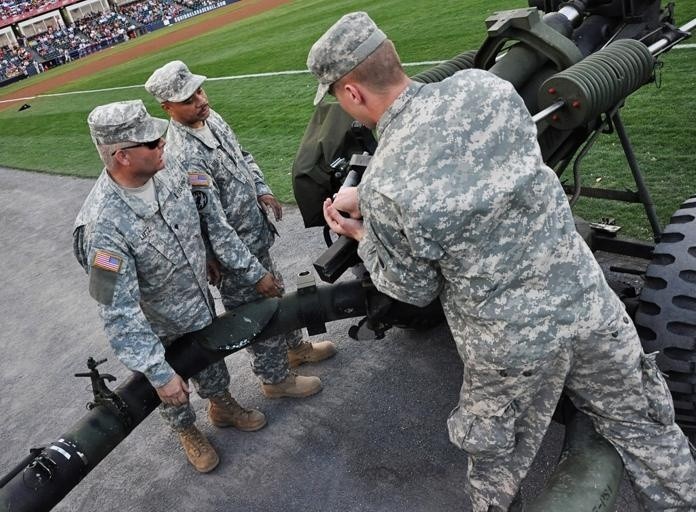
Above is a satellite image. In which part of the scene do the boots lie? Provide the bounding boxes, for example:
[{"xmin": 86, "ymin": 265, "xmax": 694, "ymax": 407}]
[
  {"xmin": 209, "ymin": 389, "xmax": 267, "ymax": 433},
  {"xmin": 287, "ymin": 339, "xmax": 337, "ymax": 368},
  {"xmin": 263, "ymin": 370, "xmax": 323, "ymax": 400},
  {"xmin": 180, "ymin": 424, "xmax": 220, "ymax": 474}
]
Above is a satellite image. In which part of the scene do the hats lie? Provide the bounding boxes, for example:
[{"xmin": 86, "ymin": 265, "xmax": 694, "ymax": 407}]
[
  {"xmin": 87, "ymin": 99, "xmax": 170, "ymax": 144},
  {"xmin": 144, "ymin": 60, "xmax": 208, "ymax": 103},
  {"xmin": 305, "ymin": 11, "xmax": 387, "ymax": 108}
]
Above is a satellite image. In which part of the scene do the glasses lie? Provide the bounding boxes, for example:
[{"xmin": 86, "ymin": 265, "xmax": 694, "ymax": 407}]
[{"xmin": 111, "ymin": 137, "xmax": 161, "ymax": 158}]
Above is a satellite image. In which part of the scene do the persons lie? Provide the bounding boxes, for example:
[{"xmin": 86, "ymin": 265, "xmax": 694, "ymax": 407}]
[
  {"xmin": 306, "ymin": 11, "xmax": 696, "ymax": 512},
  {"xmin": 72, "ymin": 99, "xmax": 267, "ymax": 473},
  {"xmin": 145, "ymin": 60, "xmax": 337, "ymax": 399},
  {"xmin": 0, "ymin": 0, "xmax": 224, "ymax": 82}
]
